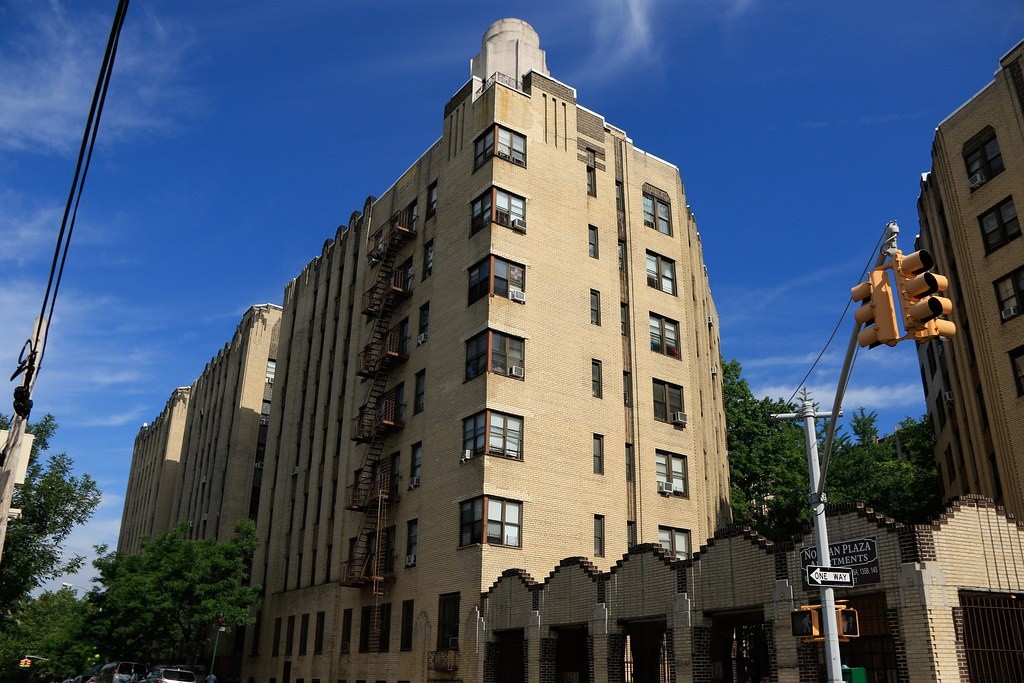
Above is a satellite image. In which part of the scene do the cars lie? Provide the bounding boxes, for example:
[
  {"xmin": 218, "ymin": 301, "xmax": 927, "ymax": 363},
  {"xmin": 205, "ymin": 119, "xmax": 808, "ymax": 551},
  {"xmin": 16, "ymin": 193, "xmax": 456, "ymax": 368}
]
[
  {"xmin": 137, "ymin": 667, "xmax": 197, "ymax": 683},
  {"xmin": 61, "ymin": 675, "xmax": 94, "ymax": 683}
]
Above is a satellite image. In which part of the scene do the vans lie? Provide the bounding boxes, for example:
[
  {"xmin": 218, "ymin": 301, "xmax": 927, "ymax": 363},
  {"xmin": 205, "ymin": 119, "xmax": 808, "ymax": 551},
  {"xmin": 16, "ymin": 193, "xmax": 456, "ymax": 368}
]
[{"xmin": 95, "ymin": 661, "xmax": 148, "ymax": 683}]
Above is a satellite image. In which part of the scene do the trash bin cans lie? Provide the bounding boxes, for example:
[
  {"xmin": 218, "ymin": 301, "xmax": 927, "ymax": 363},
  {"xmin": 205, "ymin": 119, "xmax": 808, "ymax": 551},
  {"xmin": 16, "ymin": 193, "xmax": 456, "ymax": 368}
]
[{"xmin": 842, "ymin": 668, "xmax": 867, "ymax": 683}]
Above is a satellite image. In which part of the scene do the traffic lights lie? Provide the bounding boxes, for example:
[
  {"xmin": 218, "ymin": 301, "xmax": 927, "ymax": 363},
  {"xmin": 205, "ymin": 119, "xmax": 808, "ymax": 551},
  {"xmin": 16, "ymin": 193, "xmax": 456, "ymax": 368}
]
[
  {"xmin": 27, "ymin": 660, "xmax": 31, "ymax": 667},
  {"xmin": 790, "ymin": 608, "xmax": 819, "ymax": 638},
  {"xmin": 926, "ymin": 269, "xmax": 957, "ymax": 337},
  {"xmin": 889, "ymin": 249, "xmax": 943, "ymax": 341},
  {"xmin": 19, "ymin": 660, "xmax": 25, "ymax": 667},
  {"xmin": 23, "ymin": 659, "xmax": 28, "ymax": 667},
  {"xmin": 851, "ymin": 269, "xmax": 899, "ymax": 346},
  {"xmin": 837, "ymin": 607, "xmax": 860, "ymax": 638}
]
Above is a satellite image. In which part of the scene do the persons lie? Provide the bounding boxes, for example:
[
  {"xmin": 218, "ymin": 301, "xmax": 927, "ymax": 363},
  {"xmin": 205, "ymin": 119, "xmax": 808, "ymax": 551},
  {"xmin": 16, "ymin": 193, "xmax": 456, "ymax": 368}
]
[{"xmin": 205, "ymin": 672, "xmax": 216, "ymax": 683}]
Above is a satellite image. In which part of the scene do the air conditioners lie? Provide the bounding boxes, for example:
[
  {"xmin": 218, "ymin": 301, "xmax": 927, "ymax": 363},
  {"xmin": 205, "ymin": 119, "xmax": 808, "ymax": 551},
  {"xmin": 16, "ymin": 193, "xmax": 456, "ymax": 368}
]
[
  {"xmin": 708, "ymin": 316, "xmax": 714, "ymax": 326},
  {"xmin": 511, "ymin": 290, "xmax": 526, "ymax": 302},
  {"xmin": 405, "ymin": 555, "xmax": 416, "ymax": 566},
  {"xmin": 260, "ymin": 419, "xmax": 266, "ymax": 424},
  {"xmin": 509, "ymin": 365, "xmax": 524, "ymax": 378},
  {"xmin": 201, "ymin": 513, "xmax": 208, "ymax": 520},
  {"xmin": 200, "ymin": 476, "xmax": 206, "ymax": 483},
  {"xmin": 450, "ymin": 637, "xmax": 457, "ymax": 649},
  {"xmin": 187, "ymin": 521, "xmax": 194, "ymax": 527},
  {"xmin": 511, "ymin": 219, "xmax": 527, "ymax": 230},
  {"xmin": 410, "ymin": 477, "xmax": 420, "ymax": 487},
  {"xmin": 199, "ymin": 409, "xmax": 204, "ymax": 415},
  {"xmin": 291, "ymin": 466, "xmax": 298, "ymax": 475},
  {"xmin": 712, "ymin": 366, "xmax": 717, "ymax": 375},
  {"xmin": 268, "ymin": 377, "xmax": 274, "ymax": 383},
  {"xmin": 1001, "ymin": 307, "xmax": 1018, "ymax": 319},
  {"xmin": 460, "ymin": 449, "xmax": 471, "ymax": 461},
  {"xmin": 671, "ymin": 412, "xmax": 688, "ymax": 425},
  {"xmin": 968, "ymin": 174, "xmax": 986, "ymax": 188},
  {"xmin": 417, "ymin": 333, "xmax": 427, "ymax": 344},
  {"xmin": 257, "ymin": 462, "xmax": 263, "ymax": 469},
  {"xmin": 937, "ymin": 345, "xmax": 943, "ymax": 355},
  {"xmin": 658, "ymin": 482, "xmax": 674, "ymax": 497},
  {"xmin": 943, "ymin": 391, "xmax": 953, "ymax": 403}
]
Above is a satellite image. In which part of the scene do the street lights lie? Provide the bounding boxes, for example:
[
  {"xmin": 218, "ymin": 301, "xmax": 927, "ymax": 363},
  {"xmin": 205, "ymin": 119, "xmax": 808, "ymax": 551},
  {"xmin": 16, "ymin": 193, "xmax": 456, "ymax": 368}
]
[{"xmin": 209, "ymin": 625, "xmax": 229, "ymax": 674}]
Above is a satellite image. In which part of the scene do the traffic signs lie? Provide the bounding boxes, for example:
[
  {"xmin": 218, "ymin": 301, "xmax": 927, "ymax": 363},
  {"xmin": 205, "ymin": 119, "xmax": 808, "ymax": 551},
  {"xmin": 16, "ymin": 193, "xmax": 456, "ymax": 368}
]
[{"xmin": 804, "ymin": 564, "xmax": 855, "ymax": 588}]
